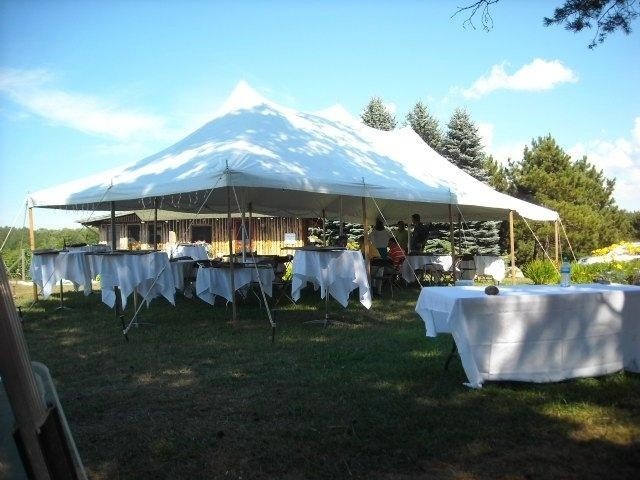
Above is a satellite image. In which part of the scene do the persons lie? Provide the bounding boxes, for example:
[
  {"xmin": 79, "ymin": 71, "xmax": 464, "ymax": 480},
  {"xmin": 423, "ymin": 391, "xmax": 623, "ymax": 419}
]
[
  {"xmin": 393, "ymin": 220, "xmax": 408, "ymax": 252},
  {"xmin": 358, "ymin": 236, "xmax": 384, "ymax": 294},
  {"xmin": 410, "ymin": 214, "xmax": 427, "ymax": 252},
  {"xmin": 384, "ymin": 237, "xmax": 406, "ymax": 275},
  {"xmin": 369, "ymin": 220, "xmax": 393, "ymax": 254}
]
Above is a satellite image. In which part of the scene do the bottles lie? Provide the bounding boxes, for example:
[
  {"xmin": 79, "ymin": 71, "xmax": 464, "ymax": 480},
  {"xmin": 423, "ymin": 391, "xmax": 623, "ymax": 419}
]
[{"xmin": 560, "ymin": 258, "xmax": 571, "ymax": 287}]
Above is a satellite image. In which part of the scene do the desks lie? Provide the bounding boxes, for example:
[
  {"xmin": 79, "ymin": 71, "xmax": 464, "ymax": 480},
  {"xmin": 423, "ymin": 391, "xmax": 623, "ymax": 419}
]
[{"xmin": 29, "ymin": 241, "xmax": 505, "ymax": 322}]
